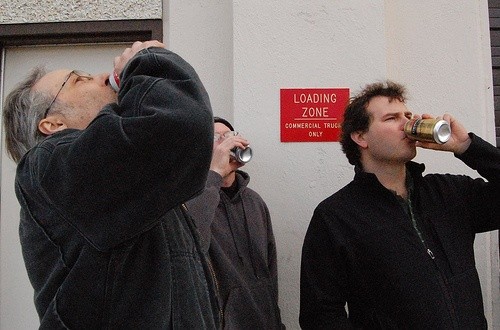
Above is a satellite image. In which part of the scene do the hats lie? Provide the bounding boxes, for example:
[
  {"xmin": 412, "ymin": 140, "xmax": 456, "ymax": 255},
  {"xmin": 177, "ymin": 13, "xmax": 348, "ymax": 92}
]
[{"xmin": 214, "ymin": 116, "xmax": 234, "ymax": 131}]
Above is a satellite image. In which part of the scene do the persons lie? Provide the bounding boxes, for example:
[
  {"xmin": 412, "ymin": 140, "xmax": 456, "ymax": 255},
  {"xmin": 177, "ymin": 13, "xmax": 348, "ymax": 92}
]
[
  {"xmin": 2, "ymin": 41, "xmax": 214, "ymax": 330},
  {"xmin": 182, "ymin": 116, "xmax": 286, "ymax": 330},
  {"xmin": 298, "ymin": 81, "xmax": 500, "ymax": 330}
]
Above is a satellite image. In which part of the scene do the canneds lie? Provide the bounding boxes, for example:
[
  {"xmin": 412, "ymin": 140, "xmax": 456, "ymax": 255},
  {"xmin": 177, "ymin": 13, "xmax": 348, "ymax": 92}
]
[
  {"xmin": 108, "ymin": 69, "xmax": 121, "ymax": 93},
  {"xmin": 229, "ymin": 145, "xmax": 253, "ymax": 164},
  {"xmin": 404, "ymin": 118, "xmax": 451, "ymax": 145}
]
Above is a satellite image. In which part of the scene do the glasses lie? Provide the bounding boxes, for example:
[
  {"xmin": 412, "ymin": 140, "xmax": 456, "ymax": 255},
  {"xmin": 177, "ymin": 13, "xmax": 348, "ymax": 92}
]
[
  {"xmin": 213, "ymin": 131, "xmax": 239, "ymax": 142},
  {"xmin": 44, "ymin": 70, "xmax": 93, "ymax": 118}
]
